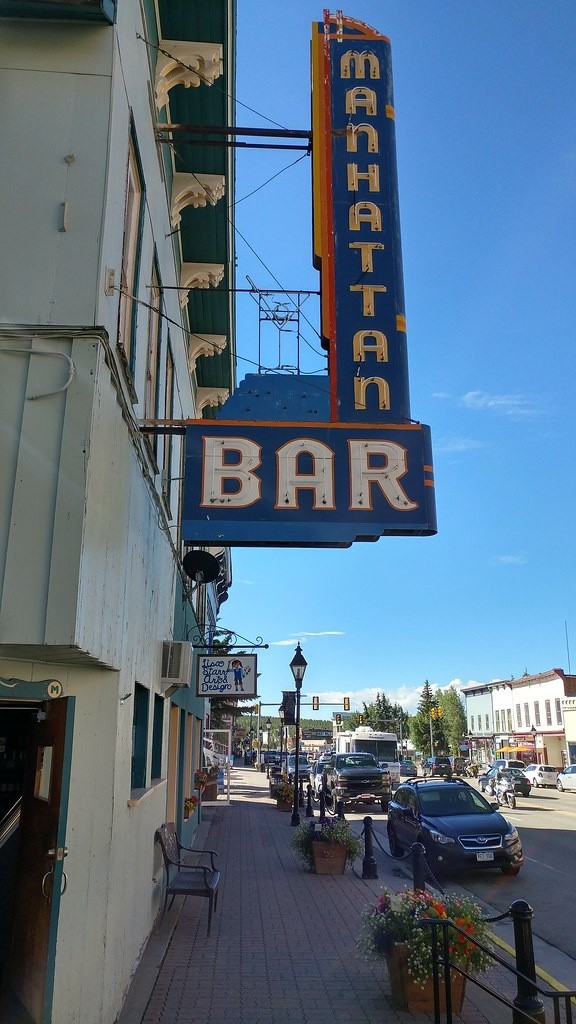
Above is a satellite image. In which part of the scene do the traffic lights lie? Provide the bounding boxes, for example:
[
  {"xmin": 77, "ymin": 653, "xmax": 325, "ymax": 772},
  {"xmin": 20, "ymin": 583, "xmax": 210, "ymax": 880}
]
[
  {"xmin": 358, "ymin": 714, "xmax": 363, "ymax": 725},
  {"xmin": 336, "ymin": 714, "xmax": 342, "ymax": 725},
  {"xmin": 431, "ymin": 705, "xmax": 443, "ymax": 719}
]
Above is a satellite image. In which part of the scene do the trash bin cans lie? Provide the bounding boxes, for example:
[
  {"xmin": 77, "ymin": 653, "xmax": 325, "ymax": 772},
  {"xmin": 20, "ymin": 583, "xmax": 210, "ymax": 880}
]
[{"xmin": 270, "ymin": 774, "xmax": 284, "ymax": 798}]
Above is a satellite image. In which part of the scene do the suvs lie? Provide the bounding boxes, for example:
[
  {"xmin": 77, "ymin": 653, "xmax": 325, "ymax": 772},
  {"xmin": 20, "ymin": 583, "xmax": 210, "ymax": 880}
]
[
  {"xmin": 321, "ymin": 753, "xmax": 392, "ymax": 812},
  {"xmin": 387, "ymin": 777, "xmax": 524, "ymax": 880},
  {"xmin": 489, "ymin": 759, "xmax": 527, "ymax": 774},
  {"xmin": 420, "ymin": 756, "xmax": 468, "ymax": 777}
]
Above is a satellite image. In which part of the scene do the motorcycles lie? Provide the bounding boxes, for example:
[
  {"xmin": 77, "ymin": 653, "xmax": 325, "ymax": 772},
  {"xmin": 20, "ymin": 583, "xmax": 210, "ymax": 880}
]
[{"xmin": 485, "ymin": 777, "xmax": 516, "ymax": 810}]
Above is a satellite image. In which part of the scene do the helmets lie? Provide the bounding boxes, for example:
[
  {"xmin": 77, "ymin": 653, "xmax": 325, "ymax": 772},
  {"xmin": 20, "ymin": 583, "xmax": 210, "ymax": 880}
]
[{"xmin": 499, "ymin": 765, "xmax": 506, "ymax": 772}]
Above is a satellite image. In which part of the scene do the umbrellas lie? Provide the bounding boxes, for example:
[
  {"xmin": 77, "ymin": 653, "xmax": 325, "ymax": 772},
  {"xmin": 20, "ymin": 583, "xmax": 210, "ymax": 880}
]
[{"xmin": 495, "ymin": 746, "xmax": 530, "ymax": 752}]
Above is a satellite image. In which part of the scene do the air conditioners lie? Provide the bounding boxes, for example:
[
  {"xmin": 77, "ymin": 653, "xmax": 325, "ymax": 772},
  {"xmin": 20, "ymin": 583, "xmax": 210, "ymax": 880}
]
[{"xmin": 159, "ymin": 641, "xmax": 193, "ymax": 687}]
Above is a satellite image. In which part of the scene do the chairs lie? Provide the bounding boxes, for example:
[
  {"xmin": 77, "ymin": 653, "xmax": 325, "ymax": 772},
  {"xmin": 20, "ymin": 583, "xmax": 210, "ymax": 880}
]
[{"xmin": 156, "ymin": 821, "xmax": 220, "ymax": 926}]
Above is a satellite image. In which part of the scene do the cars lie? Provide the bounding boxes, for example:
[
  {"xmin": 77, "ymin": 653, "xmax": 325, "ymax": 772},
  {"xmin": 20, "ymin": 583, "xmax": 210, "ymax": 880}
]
[
  {"xmin": 478, "ymin": 768, "xmax": 531, "ymax": 798},
  {"xmin": 522, "ymin": 764, "xmax": 559, "ymax": 788},
  {"xmin": 556, "ymin": 764, "xmax": 576, "ymax": 792},
  {"xmin": 247, "ymin": 748, "xmax": 338, "ymax": 798}
]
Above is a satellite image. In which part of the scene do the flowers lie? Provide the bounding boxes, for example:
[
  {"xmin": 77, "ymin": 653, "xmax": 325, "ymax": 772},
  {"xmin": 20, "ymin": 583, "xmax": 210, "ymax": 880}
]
[
  {"xmin": 206, "ymin": 766, "xmax": 219, "ymax": 782},
  {"xmin": 355, "ymin": 888, "xmax": 499, "ymax": 991},
  {"xmin": 184, "ymin": 796, "xmax": 200, "ymax": 810},
  {"xmin": 291, "ymin": 816, "xmax": 364, "ymax": 869},
  {"xmin": 272, "ymin": 783, "xmax": 295, "ymax": 803},
  {"xmin": 195, "ymin": 769, "xmax": 207, "ymax": 791}
]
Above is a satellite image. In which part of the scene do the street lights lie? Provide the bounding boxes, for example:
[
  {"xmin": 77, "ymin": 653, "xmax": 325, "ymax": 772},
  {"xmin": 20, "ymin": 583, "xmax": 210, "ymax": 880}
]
[
  {"xmin": 278, "ymin": 703, "xmax": 284, "ymax": 774},
  {"xmin": 530, "ymin": 725, "xmax": 537, "ymax": 764},
  {"xmin": 400, "ymin": 760, "xmax": 417, "ymax": 777},
  {"xmin": 289, "ymin": 642, "xmax": 308, "ymax": 826},
  {"xmin": 265, "ymin": 718, "xmax": 272, "ymax": 780},
  {"xmin": 468, "ymin": 730, "xmax": 473, "ymax": 762}
]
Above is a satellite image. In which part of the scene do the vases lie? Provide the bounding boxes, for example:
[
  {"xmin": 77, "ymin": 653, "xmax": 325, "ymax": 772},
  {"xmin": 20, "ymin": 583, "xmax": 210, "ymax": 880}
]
[
  {"xmin": 201, "ymin": 786, "xmax": 206, "ymax": 793},
  {"xmin": 206, "ymin": 780, "xmax": 216, "ymax": 786},
  {"xmin": 278, "ymin": 797, "xmax": 292, "ymax": 812},
  {"xmin": 185, "ymin": 808, "xmax": 194, "ymax": 819},
  {"xmin": 385, "ymin": 944, "xmax": 468, "ymax": 1013},
  {"xmin": 312, "ymin": 841, "xmax": 350, "ymax": 874}
]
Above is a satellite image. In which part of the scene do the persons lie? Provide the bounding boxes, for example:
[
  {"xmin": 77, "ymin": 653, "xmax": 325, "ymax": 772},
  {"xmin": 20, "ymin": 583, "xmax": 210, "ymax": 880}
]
[
  {"xmin": 233, "ymin": 747, "xmax": 240, "ymax": 760},
  {"xmin": 495, "ymin": 765, "xmax": 515, "ymax": 785}
]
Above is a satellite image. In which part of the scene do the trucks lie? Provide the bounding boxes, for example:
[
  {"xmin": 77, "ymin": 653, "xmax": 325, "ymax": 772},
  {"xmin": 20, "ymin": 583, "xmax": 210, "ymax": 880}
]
[{"xmin": 335, "ymin": 728, "xmax": 400, "ymax": 785}]
